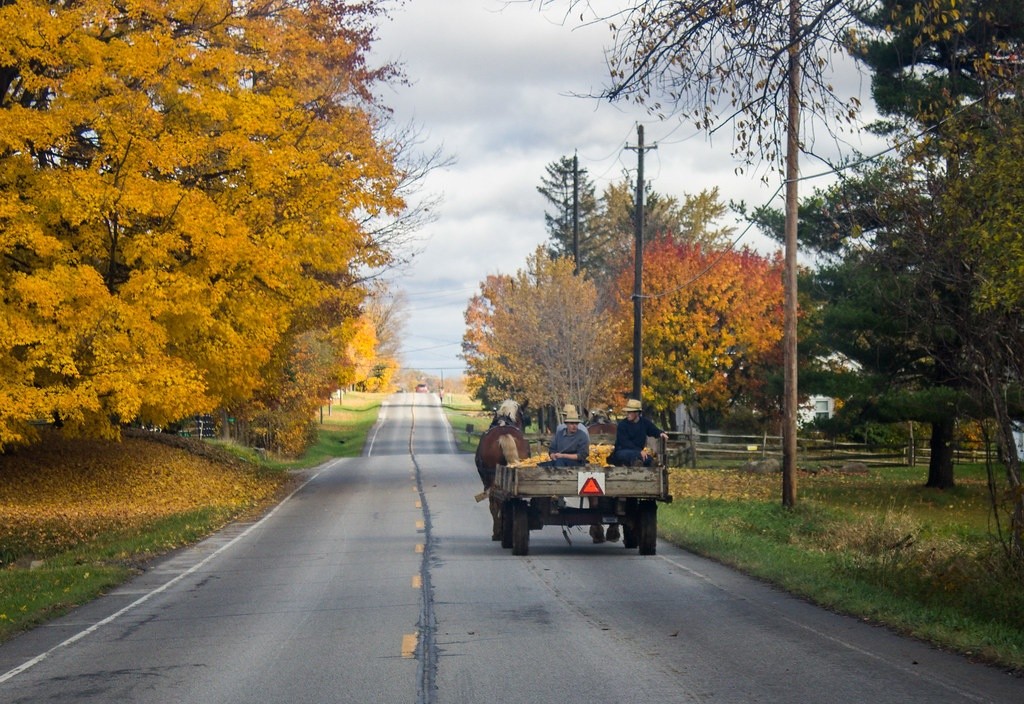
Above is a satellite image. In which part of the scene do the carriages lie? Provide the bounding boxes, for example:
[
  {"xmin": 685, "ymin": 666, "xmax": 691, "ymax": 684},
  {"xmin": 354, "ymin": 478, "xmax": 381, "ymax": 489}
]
[{"xmin": 474, "ymin": 398, "xmax": 675, "ymax": 556}]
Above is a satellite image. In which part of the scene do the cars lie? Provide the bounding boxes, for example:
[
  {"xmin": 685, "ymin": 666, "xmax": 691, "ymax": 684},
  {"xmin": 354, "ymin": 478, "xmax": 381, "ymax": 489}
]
[{"xmin": 416, "ymin": 383, "xmax": 428, "ymax": 394}]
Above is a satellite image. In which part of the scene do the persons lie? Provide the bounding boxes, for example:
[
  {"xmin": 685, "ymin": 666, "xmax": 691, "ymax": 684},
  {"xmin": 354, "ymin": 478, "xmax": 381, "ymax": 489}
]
[
  {"xmin": 613, "ymin": 399, "xmax": 669, "ymax": 466},
  {"xmin": 537, "ymin": 403, "xmax": 590, "ymax": 467}
]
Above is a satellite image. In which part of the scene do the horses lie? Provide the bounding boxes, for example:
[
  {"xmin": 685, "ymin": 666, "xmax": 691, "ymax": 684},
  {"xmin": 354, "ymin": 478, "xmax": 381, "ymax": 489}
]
[
  {"xmin": 474, "ymin": 398, "xmax": 532, "ymax": 542},
  {"xmin": 585, "ymin": 408, "xmax": 620, "ymax": 544}
]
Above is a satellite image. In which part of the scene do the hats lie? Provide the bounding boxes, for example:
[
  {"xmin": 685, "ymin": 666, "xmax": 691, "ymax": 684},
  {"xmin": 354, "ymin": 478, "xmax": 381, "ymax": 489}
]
[
  {"xmin": 558, "ymin": 405, "xmax": 575, "ymax": 415},
  {"xmin": 564, "ymin": 410, "xmax": 581, "ymax": 423},
  {"xmin": 621, "ymin": 399, "xmax": 642, "ymax": 411}
]
